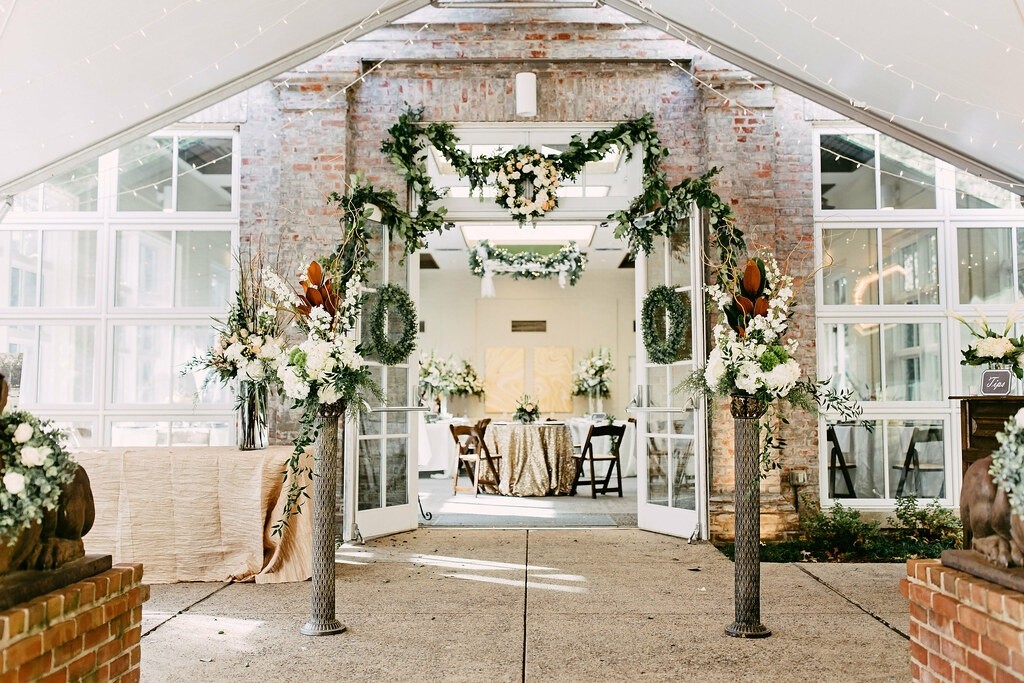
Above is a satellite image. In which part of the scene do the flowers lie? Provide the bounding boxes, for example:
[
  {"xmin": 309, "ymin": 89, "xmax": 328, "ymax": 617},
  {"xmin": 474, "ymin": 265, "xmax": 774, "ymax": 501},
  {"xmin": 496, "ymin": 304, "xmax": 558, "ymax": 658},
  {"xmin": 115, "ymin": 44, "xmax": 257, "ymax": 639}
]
[
  {"xmin": 570, "ymin": 347, "xmax": 618, "ymax": 401},
  {"xmin": 514, "ymin": 394, "xmax": 542, "ymax": 420},
  {"xmin": 685, "ymin": 225, "xmax": 873, "ymax": 432},
  {"xmin": 950, "ymin": 304, "xmax": 1023, "ymax": 395},
  {"xmin": 4, "ymin": 372, "xmax": 78, "ymax": 549},
  {"xmin": 417, "ymin": 353, "xmax": 487, "ymax": 404},
  {"xmin": 180, "ymin": 259, "xmax": 293, "ymax": 405},
  {"xmin": 493, "ymin": 145, "xmax": 563, "ymax": 223},
  {"xmin": 260, "ymin": 258, "xmax": 394, "ymax": 417}
]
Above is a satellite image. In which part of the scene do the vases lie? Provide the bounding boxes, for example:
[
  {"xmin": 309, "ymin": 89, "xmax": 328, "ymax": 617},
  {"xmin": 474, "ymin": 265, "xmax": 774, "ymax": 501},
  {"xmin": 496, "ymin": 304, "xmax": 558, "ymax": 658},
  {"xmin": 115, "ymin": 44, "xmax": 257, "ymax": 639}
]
[
  {"xmin": 235, "ymin": 355, "xmax": 277, "ymax": 457},
  {"xmin": 298, "ymin": 407, "xmax": 345, "ymax": 639},
  {"xmin": 521, "ymin": 413, "xmax": 537, "ymax": 424},
  {"xmin": 728, "ymin": 392, "xmax": 773, "ymax": 641},
  {"xmin": 435, "ymin": 389, "xmax": 458, "ymax": 417},
  {"xmin": 587, "ymin": 386, "xmax": 606, "ymax": 418}
]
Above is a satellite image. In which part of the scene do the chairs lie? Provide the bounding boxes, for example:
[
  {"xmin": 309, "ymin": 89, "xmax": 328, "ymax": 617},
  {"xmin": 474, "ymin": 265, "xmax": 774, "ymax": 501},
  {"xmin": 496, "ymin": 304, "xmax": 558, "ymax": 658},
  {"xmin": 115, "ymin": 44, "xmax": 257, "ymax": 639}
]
[
  {"xmin": 568, "ymin": 422, "xmax": 627, "ymax": 498},
  {"xmin": 889, "ymin": 424, "xmax": 946, "ymax": 498},
  {"xmin": 825, "ymin": 426, "xmax": 855, "ymax": 498},
  {"xmin": 448, "ymin": 418, "xmax": 501, "ymax": 494},
  {"xmin": 628, "ymin": 417, "xmax": 682, "ymax": 494}
]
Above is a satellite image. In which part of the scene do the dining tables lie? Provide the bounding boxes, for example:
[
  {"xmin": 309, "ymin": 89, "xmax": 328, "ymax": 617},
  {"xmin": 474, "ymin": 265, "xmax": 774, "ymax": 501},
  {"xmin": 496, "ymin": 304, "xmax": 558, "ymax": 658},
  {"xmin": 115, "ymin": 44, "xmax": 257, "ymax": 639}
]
[{"xmin": 479, "ymin": 421, "xmax": 577, "ymax": 497}]
